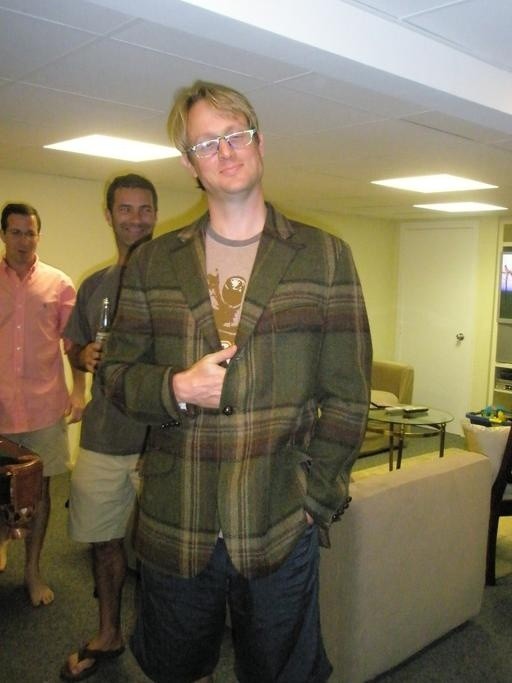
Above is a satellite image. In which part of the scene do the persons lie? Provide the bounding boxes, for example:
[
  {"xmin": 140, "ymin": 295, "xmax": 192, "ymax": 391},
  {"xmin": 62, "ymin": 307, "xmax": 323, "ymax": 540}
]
[
  {"xmin": 95, "ymin": 78, "xmax": 373, "ymax": 683},
  {"xmin": 62, "ymin": 172, "xmax": 159, "ymax": 681},
  {"xmin": 1, "ymin": 203, "xmax": 86, "ymax": 606}
]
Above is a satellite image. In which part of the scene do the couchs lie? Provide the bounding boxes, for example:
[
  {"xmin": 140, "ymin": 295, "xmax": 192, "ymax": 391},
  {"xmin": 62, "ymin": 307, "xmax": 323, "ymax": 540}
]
[
  {"xmin": 319, "ymin": 449, "xmax": 490, "ymax": 683},
  {"xmin": 358, "ymin": 362, "xmax": 415, "ymax": 458},
  {"xmin": 59, "ymin": 339, "xmax": 92, "ymax": 471}
]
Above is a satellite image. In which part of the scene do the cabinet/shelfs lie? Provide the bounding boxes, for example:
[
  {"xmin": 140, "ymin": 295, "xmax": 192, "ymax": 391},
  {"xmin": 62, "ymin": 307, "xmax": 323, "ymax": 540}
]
[{"xmin": 488, "ymin": 218, "xmax": 512, "ymax": 411}]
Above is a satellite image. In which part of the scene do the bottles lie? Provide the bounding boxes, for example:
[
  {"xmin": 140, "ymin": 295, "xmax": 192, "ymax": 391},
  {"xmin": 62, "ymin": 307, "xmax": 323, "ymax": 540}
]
[{"xmin": 93, "ymin": 298, "xmax": 114, "ymax": 348}]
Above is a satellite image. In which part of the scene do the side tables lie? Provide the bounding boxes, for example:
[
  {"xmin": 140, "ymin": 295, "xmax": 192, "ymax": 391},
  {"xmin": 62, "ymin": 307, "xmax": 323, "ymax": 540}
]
[{"xmin": 466, "ymin": 411, "xmax": 511, "ymax": 426}]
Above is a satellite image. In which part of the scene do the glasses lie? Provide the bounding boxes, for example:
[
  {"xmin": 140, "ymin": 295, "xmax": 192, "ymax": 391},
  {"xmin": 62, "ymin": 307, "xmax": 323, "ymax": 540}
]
[{"xmin": 185, "ymin": 130, "xmax": 255, "ymax": 159}]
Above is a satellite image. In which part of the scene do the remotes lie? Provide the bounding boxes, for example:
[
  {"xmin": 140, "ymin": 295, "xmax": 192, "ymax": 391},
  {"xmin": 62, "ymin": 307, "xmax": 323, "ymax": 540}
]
[
  {"xmin": 385, "ymin": 406, "xmax": 411, "ymax": 411},
  {"xmin": 403, "ymin": 407, "xmax": 429, "ymax": 412}
]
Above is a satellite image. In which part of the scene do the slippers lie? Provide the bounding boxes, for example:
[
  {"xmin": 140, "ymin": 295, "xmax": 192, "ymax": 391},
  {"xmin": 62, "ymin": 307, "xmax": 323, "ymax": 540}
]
[{"xmin": 58, "ymin": 639, "xmax": 125, "ymax": 678}]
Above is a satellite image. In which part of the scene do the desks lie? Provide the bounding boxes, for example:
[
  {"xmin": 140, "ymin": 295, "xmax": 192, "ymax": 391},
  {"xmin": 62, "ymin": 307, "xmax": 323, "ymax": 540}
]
[{"xmin": 0, "ymin": 433, "xmax": 47, "ymax": 543}]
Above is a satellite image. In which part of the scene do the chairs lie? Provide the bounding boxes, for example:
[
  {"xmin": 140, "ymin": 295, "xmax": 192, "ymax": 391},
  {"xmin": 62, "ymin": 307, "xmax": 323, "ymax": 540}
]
[{"xmin": 485, "ymin": 429, "xmax": 511, "ymax": 586}]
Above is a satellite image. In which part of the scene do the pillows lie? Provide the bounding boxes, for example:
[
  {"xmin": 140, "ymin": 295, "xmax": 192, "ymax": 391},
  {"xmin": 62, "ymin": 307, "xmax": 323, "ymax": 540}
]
[{"xmin": 462, "ymin": 421, "xmax": 510, "ymax": 483}]
